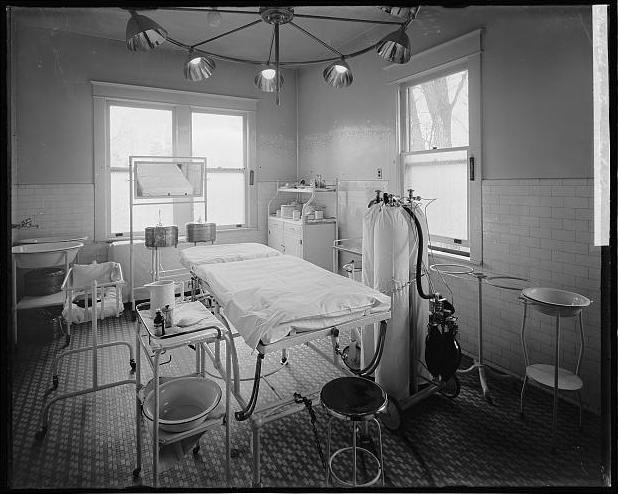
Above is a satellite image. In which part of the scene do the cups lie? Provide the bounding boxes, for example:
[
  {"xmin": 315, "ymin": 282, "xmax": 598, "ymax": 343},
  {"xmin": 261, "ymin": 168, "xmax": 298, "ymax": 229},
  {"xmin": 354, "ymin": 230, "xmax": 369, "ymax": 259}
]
[{"xmin": 275, "ymin": 203, "xmax": 324, "ymax": 221}]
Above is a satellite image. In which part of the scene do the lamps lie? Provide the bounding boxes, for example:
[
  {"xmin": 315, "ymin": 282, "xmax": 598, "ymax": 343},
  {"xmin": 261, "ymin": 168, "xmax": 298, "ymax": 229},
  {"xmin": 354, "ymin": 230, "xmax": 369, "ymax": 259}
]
[{"xmin": 114, "ymin": 0, "xmax": 422, "ymax": 106}]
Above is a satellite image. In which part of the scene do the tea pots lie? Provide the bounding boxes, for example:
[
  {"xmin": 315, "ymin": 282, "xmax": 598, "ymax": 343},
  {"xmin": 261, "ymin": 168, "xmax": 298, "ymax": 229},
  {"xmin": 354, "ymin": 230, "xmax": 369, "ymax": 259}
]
[{"xmin": 143, "ymin": 279, "xmax": 185, "ymax": 320}]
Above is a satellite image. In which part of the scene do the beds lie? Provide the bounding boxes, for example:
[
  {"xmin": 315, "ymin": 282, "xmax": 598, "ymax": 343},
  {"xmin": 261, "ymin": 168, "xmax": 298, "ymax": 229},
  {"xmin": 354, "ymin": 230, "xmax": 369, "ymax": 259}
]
[{"xmin": 178, "ymin": 240, "xmax": 394, "ymax": 486}]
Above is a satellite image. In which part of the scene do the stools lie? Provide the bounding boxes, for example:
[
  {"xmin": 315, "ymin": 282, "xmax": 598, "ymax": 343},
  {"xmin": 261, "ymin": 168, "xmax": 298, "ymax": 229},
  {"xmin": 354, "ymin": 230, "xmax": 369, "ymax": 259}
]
[{"xmin": 318, "ymin": 374, "xmax": 391, "ymax": 486}]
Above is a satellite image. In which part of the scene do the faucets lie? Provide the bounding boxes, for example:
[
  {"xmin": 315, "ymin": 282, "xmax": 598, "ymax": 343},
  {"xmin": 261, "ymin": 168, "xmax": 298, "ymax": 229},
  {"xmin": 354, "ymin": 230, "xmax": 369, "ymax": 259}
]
[{"xmin": 11, "ymin": 217, "xmax": 41, "ymax": 231}]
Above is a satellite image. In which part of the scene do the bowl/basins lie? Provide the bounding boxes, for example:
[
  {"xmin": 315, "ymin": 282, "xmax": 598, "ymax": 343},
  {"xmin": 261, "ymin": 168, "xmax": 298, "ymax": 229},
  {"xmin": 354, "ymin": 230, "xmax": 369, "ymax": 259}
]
[
  {"xmin": 23, "ymin": 268, "xmax": 66, "ymax": 296},
  {"xmin": 12, "ymin": 240, "xmax": 84, "ymax": 269},
  {"xmin": 521, "ymin": 286, "xmax": 590, "ymax": 317},
  {"xmin": 141, "ymin": 376, "xmax": 224, "ymax": 433},
  {"xmin": 16, "ymin": 234, "xmax": 90, "ymax": 244}
]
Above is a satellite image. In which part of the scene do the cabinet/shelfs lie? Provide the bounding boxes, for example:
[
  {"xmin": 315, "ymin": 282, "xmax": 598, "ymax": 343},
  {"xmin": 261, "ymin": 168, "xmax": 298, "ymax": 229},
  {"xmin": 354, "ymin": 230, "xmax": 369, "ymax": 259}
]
[
  {"xmin": 13, "ymin": 238, "xmax": 82, "ymax": 347},
  {"xmin": 266, "ymin": 176, "xmax": 341, "ymax": 272},
  {"xmin": 33, "ymin": 260, "xmax": 146, "ymax": 443},
  {"xmin": 130, "ymin": 299, "xmax": 236, "ymax": 487}
]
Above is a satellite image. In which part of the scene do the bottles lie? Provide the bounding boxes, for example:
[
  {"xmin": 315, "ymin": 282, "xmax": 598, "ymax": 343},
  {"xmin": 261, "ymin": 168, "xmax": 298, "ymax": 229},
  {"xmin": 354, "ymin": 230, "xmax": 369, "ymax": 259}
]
[
  {"xmin": 162, "ymin": 304, "xmax": 174, "ymax": 327},
  {"xmin": 153, "ymin": 308, "xmax": 166, "ymax": 336}
]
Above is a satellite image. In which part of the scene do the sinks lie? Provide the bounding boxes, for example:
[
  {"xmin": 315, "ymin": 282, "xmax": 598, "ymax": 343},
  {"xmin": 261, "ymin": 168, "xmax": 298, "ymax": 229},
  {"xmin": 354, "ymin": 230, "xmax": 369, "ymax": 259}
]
[
  {"xmin": 17, "ymin": 235, "xmax": 88, "ymax": 243},
  {"xmin": 10, "ymin": 243, "xmax": 85, "ymax": 271}
]
